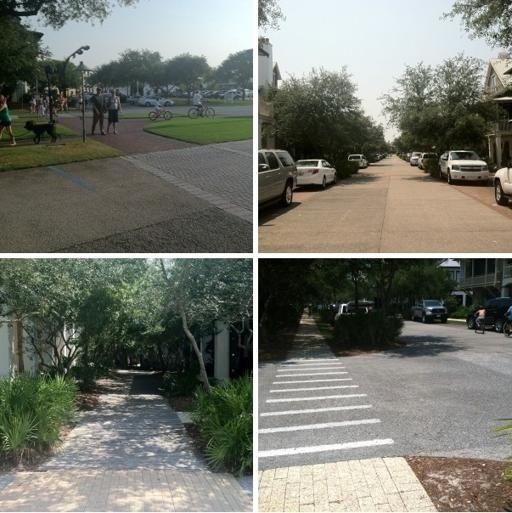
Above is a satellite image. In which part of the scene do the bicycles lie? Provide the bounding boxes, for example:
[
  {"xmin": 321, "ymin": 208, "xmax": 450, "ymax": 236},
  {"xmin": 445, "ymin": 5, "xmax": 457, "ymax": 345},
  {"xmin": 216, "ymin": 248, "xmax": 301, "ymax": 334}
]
[
  {"xmin": 147, "ymin": 108, "xmax": 172, "ymax": 122},
  {"xmin": 187, "ymin": 102, "xmax": 216, "ymax": 121},
  {"xmin": 503, "ymin": 312, "xmax": 512, "ymax": 336},
  {"xmin": 474, "ymin": 315, "xmax": 485, "ymax": 334}
]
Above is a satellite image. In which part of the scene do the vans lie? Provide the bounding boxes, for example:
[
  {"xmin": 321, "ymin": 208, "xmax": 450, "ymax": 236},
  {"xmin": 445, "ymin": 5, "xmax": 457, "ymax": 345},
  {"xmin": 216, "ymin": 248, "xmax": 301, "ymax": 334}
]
[{"xmin": 467, "ymin": 296, "xmax": 511, "ymax": 332}]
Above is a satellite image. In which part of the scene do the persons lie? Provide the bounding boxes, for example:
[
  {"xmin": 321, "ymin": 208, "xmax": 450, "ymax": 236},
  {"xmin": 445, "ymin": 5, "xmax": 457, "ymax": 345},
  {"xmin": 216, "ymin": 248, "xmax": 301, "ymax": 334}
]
[
  {"xmin": 193, "ymin": 90, "xmax": 203, "ymax": 116},
  {"xmin": 474, "ymin": 305, "xmax": 486, "ymax": 327},
  {"xmin": 29, "ymin": 91, "xmax": 82, "ymax": 117},
  {"xmin": 0, "ymin": 94, "xmax": 17, "ymax": 146},
  {"xmin": 107, "ymin": 89, "xmax": 123, "ymax": 135},
  {"xmin": 90, "ymin": 88, "xmax": 107, "ymax": 135},
  {"xmin": 503, "ymin": 303, "xmax": 512, "ymax": 335},
  {"xmin": 154, "ymin": 98, "xmax": 162, "ymax": 116}
]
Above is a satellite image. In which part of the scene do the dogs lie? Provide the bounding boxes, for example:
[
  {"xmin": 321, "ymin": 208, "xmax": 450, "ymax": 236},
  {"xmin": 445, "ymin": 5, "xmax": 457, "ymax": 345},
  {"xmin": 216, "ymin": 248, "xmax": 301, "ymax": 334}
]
[{"xmin": 23, "ymin": 119, "xmax": 57, "ymax": 145}]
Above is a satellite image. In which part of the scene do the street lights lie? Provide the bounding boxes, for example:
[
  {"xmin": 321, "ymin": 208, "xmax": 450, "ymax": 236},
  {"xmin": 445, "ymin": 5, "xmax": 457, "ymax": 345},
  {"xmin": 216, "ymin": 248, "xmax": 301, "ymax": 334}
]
[
  {"xmin": 73, "ymin": 61, "xmax": 93, "ymax": 142},
  {"xmin": 62, "ymin": 44, "xmax": 89, "ymax": 109}
]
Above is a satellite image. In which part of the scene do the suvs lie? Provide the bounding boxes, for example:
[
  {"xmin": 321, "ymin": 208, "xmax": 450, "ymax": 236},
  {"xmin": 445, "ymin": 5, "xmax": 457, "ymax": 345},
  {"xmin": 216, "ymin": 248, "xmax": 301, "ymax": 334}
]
[
  {"xmin": 439, "ymin": 149, "xmax": 489, "ymax": 184},
  {"xmin": 258, "ymin": 149, "xmax": 298, "ymax": 209}
]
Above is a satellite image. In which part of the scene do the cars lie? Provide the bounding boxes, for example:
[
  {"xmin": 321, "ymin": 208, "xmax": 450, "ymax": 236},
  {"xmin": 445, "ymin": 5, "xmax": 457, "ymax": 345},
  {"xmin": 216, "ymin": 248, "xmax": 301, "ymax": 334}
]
[
  {"xmin": 494, "ymin": 158, "xmax": 512, "ymax": 204},
  {"xmin": 409, "ymin": 299, "xmax": 448, "ymax": 324},
  {"xmin": 375, "ymin": 151, "xmax": 388, "ymax": 160},
  {"xmin": 418, "ymin": 152, "xmax": 437, "ymax": 168},
  {"xmin": 348, "ymin": 153, "xmax": 368, "ymax": 169},
  {"xmin": 397, "ymin": 151, "xmax": 411, "ymax": 161},
  {"xmin": 410, "ymin": 152, "xmax": 423, "ymax": 165},
  {"xmin": 294, "ymin": 159, "xmax": 339, "ymax": 187},
  {"xmin": 182, "ymin": 88, "xmax": 253, "ymax": 101},
  {"xmin": 84, "ymin": 90, "xmax": 127, "ymax": 105},
  {"xmin": 138, "ymin": 95, "xmax": 175, "ymax": 107},
  {"xmin": 318, "ymin": 301, "xmax": 374, "ymax": 323},
  {"xmin": 127, "ymin": 95, "xmax": 146, "ymax": 104}
]
